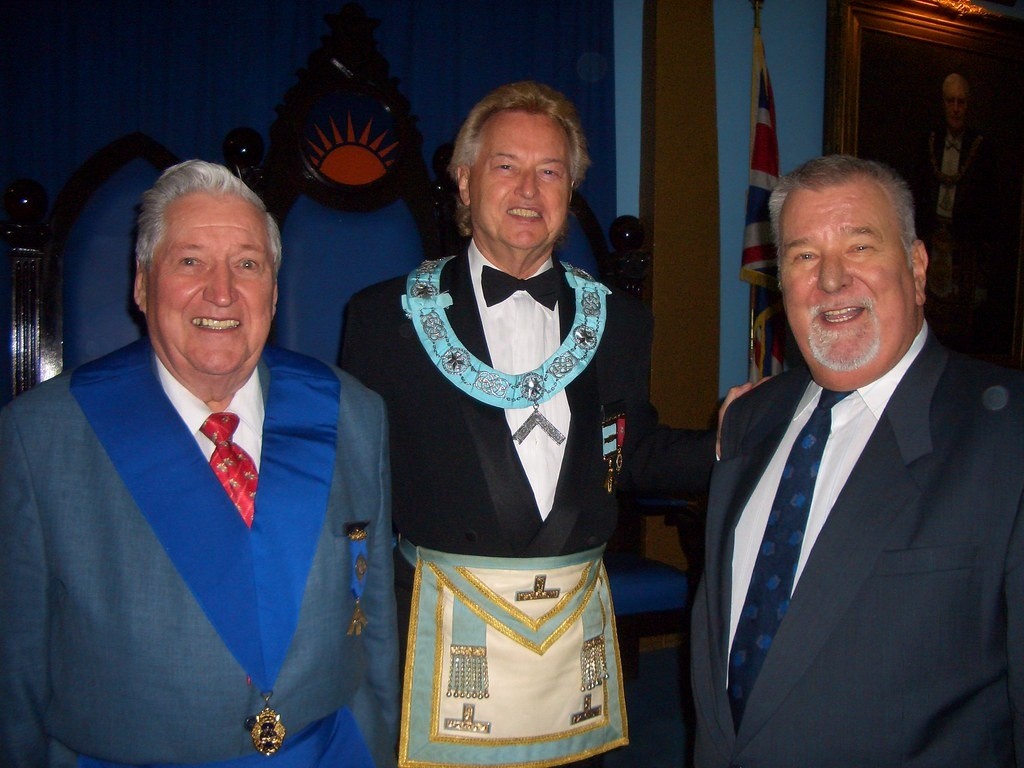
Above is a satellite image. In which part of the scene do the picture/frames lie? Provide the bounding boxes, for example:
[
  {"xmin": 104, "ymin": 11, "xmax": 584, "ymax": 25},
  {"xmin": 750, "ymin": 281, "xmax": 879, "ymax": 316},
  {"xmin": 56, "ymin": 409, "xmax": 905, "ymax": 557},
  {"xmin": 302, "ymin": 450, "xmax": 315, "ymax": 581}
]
[{"xmin": 821, "ymin": 0, "xmax": 1024, "ymax": 370}]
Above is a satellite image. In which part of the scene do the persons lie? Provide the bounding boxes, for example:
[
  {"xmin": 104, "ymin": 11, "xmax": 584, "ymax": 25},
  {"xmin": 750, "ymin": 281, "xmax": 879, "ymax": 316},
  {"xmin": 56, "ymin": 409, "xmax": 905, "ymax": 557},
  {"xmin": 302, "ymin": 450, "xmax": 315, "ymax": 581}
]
[
  {"xmin": 693, "ymin": 154, "xmax": 1024, "ymax": 768},
  {"xmin": 0, "ymin": 159, "xmax": 400, "ymax": 768},
  {"xmin": 339, "ymin": 83, "xmax": 773, "ymax": 767},
  {"xmin": 912, "ymin": 73, "xmax": 998, "ymax": 309}
]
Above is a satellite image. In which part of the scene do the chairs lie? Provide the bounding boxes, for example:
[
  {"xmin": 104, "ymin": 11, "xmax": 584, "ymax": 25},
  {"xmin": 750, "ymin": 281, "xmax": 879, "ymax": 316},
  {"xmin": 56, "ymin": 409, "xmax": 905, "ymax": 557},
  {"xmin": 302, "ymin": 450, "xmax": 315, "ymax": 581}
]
[{"xmin": 438, "ymin": 147, "xmax": 705, "ymax": 641}]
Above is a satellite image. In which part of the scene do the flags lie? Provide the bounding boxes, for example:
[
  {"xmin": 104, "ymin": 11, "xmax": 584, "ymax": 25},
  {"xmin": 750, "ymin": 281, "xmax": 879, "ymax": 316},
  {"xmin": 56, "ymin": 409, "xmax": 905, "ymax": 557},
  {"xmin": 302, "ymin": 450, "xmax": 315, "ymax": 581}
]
[{"xmin": 741, "ymin": 38, "xmax": 780, "ymax": 378}]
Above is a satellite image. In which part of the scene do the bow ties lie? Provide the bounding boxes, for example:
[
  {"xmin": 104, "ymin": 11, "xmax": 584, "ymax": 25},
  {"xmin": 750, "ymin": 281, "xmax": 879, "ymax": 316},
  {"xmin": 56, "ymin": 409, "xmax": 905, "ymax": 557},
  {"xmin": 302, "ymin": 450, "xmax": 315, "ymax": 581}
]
[
  {"xmin": 482, "ymin": 265, "xmax": 560, "ymax": 311},
  {"xmin": 946, "ymin": 141, "xmax": 960, "ymax": 151}
]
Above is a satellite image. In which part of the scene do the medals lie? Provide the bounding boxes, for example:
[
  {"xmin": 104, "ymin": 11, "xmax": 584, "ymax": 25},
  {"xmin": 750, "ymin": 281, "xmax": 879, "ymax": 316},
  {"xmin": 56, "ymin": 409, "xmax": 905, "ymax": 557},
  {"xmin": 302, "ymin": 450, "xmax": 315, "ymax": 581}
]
[{"xmin": 249, "ymin": 707, "xmax": 285, "ymax": 756}]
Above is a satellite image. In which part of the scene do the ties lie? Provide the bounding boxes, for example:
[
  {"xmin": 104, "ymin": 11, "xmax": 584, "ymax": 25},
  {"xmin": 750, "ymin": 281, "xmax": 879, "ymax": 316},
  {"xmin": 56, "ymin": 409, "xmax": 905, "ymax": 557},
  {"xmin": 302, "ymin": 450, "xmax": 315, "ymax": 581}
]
[
  {"xmin": 200, "ymin": 412, "xmax": 258, "ymax": 528},
  {"xmin": 728, "ymin": 387, "xmax": 859, "ymax": 740}
]
